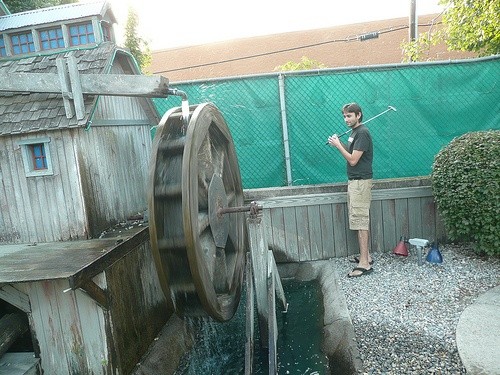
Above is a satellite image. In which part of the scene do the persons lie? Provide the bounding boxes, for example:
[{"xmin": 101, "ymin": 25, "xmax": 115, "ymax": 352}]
[{"xmin": 328, "ymin": 102, "xmax": 377, "ymax": 279}]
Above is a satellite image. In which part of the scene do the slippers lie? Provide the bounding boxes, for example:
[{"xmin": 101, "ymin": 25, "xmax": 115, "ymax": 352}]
[
  {"xmin": 348, "ymin": 267, "xmax": 373, "ymax": 278},
  {"xmin": 349, "ymin": 256, "xmax": 374, "ymax": 264}
]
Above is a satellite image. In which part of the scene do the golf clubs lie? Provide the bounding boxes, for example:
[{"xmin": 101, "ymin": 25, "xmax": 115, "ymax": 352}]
[{"xmin": 326, "ymin": 106, "xmax": 397, "ymax": 145}]
